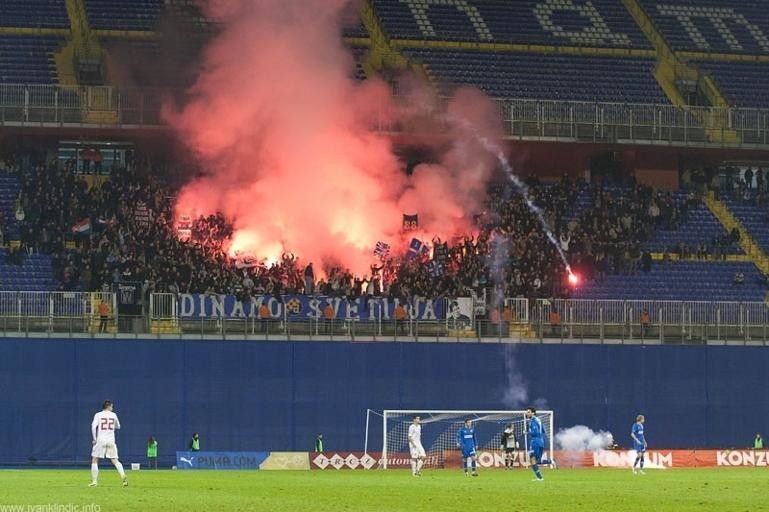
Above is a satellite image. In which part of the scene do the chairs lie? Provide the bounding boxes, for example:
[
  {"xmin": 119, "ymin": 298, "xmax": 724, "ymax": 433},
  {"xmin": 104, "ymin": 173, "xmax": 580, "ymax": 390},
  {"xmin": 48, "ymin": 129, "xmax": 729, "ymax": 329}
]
[{"xmin": 0, "ymin": 0, "xmax": 769, "ymax": 325}]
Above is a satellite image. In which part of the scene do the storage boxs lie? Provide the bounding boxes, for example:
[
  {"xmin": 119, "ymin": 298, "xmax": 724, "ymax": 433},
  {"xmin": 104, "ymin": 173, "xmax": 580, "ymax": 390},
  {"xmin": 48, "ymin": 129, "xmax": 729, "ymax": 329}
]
[{"xmin": 131, "ymin": 463, "xmax": 140, "ymax": 470}]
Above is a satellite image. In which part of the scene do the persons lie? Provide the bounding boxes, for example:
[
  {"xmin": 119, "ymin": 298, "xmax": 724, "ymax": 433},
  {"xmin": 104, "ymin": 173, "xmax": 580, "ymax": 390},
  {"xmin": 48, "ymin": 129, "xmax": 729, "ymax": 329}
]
[
  {"xmin": 752, "ymin": 431, "xmax": 768, "ymax": 449},
  {"xmin": 261, "ymin": 302, "xmax": 272, "ymax": 333},
  {"xmin": 391, "ymin": 303, "xmax": 406, "ymax": 334},
  {"xmin": 500, "ymin": 422, "xmax": 520, "ymax": 470},
  {"xmin": 407, "ymin": 415, "xmax": 427, "ymax": 476},
  {"xmin": 312, "ymin": 430, "xmax": 324, "ymax": 452},
  {"xmin": 640, "ymin": 308, "xmax": 650, "ymax": 337},
  {"xmin": 522, "ymin": 406, "xmax": 559, "ymax": 482},
  {"xmin": 456, "ymin": 418, "xmax": 480, "ymax": 477},
  {"xmin": 550, "ymin": 307, "xmax": 560, "ymax": 335},
  {"xmin": 628, "ymin": 413, "xmax": 649, "ymax": 476},
  {"xmin": 323, "ymin": 303, "xmax": 335, "ymax": 335},
  {"xmin": 189, "ymin": 431, "xmax": 201, "ymax": 452},
  {"xmin": 1, "ymin": 141, "xmax": 769, "ymax": 307},
  {"xmin": 98, "ymin": 299, "xmax": 111, "ymax": 333},
  {"xmin": 500, "ymin": 306, "xmax": 512, "ymax": 338},
  {"xmin": 89, "ymin": 399, "xmax": 129, "ymax": 488},
  {"xmin": 490, "ymin": 307, "xmax": 500, "ymax": 334},
  {"xmin": 146, "ymin": 433, "xmax": 160, "ymax": 469}
]
